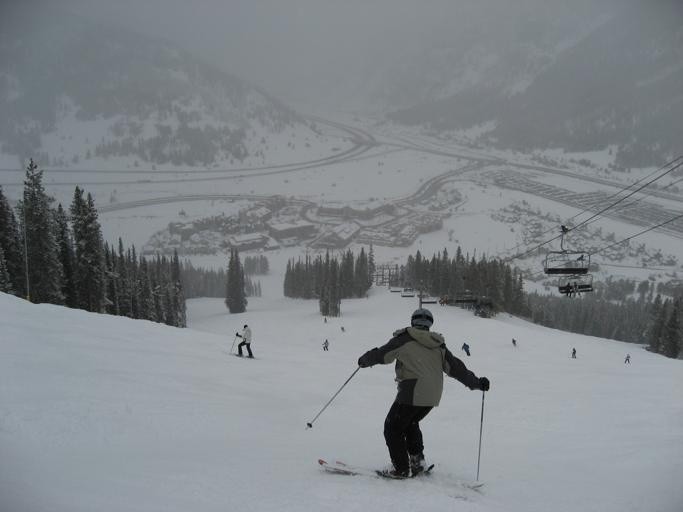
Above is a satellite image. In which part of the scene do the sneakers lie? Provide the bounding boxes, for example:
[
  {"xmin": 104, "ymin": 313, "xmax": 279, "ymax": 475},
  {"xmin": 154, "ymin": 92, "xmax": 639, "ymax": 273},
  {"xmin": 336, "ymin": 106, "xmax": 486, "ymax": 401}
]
[
  {"xmin": 236, "ymin": 354, "xmax": 241, "ymax": 357},
  {"xmin": 249, "ymin": 355, "xmax": 254, "ymax": 358}
]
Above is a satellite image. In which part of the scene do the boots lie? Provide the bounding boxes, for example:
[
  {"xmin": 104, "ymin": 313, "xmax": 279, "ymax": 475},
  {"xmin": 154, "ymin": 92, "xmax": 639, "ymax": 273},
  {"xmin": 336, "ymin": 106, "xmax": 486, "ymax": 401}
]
[
  {"xmin": 389, "ymin": 463, "xmax": 410, "ymax": 478},
  {"xmin": 410, "ymin": 454, "xmax": 426, "ymax": 473}
]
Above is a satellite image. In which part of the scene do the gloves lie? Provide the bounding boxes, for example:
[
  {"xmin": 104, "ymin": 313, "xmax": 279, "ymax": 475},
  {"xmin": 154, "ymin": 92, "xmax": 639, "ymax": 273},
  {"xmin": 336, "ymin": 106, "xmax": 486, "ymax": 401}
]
[
  {"xmin": 477, "ymin": 377, "xmax": 490, "ymax": 391},
  {"xmin": 236, "ymin": 332, "xmax": 238, "ymax": 336},
  {"xmin": 357, "ymin": 352, "xmax": 372, "ymax": 368}
]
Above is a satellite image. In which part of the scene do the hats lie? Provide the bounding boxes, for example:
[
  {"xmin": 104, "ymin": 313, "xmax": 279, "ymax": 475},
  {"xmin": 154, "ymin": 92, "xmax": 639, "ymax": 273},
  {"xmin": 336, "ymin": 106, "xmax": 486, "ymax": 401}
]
[{"xmin": 244, "ymin": 325, "xmax": 248, "ymax": 328}]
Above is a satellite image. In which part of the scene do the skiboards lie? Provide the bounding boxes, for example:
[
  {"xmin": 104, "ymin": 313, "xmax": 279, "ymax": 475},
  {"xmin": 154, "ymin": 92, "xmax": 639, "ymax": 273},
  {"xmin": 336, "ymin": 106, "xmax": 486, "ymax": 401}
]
[
  {"xmin": 318, "ymin": 460, "xmax": 484, "ymax": 489},
  {"xmin": 228, "ymin": 353, "xmax": 257, "ymax": 359}
]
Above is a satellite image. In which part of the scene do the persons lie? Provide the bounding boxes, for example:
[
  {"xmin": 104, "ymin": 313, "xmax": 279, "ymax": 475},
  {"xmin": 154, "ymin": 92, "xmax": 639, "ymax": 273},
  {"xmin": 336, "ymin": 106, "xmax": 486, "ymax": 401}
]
[
  {"xmin": 572, "ymin": 282, "xmax": 581, "ymax": 299},
  {"xmin": 460, "ymin": 342, "xmax": 470, "ymax": 356},
  {"xmin": 623, "ymin": 353, "xmax": 630, "ymax": 364},
  {"xmin": 512, "ymin": 338, "xmax": 517, "ymax": 346},
  {"xmin": 357, "ymin": 308, "xmax": 490, "ymax": 481},
  {"xmin": 321, "ymin": 339, "xmax": 329, "ymax": 351},
  {"xmin": 235, "ymin": 324, "xmax": 252, "ymax": 358},
  {"xmin": 572, "ymin": 347, "xmax": 576, "ymax": 358},
  {"xmin": 564, "ymin": 282, "xmax": 573, "ymax": 297}
]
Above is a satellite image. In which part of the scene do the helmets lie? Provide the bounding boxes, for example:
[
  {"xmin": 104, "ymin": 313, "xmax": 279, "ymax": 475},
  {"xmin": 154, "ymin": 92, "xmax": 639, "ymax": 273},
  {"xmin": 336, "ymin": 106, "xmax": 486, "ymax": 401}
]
[{"xmin": 412, "ymin": 308, "xmax": 433, "ymax": 327}]
